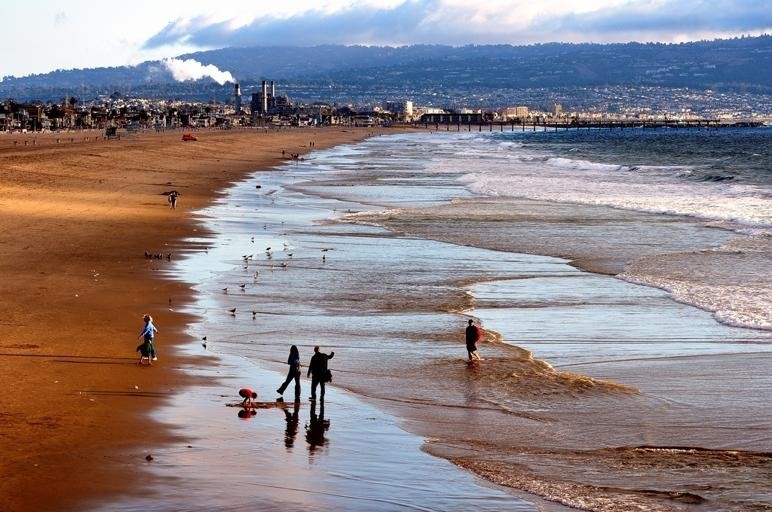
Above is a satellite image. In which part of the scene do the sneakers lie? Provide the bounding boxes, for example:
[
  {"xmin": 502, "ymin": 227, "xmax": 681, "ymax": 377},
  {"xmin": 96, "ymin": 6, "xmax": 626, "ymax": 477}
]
[{"xmin": 143, "ymin": 356, "xmax": 158, "ymax": 361}]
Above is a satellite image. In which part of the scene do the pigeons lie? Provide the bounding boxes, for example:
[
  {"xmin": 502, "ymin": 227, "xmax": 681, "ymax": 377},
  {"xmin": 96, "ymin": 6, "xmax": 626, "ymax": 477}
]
[
  {"xmin": 228, "ymin": 307, "xmax": 236, "ymax": 314},
  {"xmin": 252, "ymin": 311, "xmax": 256, "ymax": 315},
  {"xmin": 202, "ymin": 336, "xmax": 207, "ymax": 343},
  {"xmin": 242, "ymin": 243, "xmax": 294, "ymax": 279},
  {"xmin": 240, "ymin": 284, "xmax": 245, "ymax": 289},
  {"xmin": 143, "ymin": 250, "xmax": 172, "ymax": 261},
  {"xmin": 221, "ymin": 287, "xmax": 227, "ymax": 291}
]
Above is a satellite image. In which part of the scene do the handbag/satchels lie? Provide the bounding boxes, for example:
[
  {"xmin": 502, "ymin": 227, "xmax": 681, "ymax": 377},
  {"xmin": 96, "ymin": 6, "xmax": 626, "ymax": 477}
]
[{"xmin": 323, "ymin": 369, "xmax": 332, "ymax": 383}]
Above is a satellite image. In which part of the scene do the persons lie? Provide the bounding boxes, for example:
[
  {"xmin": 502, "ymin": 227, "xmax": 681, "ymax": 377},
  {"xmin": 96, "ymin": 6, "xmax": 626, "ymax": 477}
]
[
  {"xmin": 465, "ymin": 318, "xmax": 483, "ymax": 361},
  {"xmin": 137, "ymin": 334, "xmax": 153, "ymax": 366},
  {"xmin": 167, "ymin": 192, "xmax": 174, "ymax": 210},
  {"xmin": 137, "ymin": 314, "xmax": 159, "ymax": 361},
  {"xmin": 306, "ymin": 345, "xmax": 335, "ymax": 402},
  {"xmin": 170, "ymin": 190, "xmax": 179, "ymax": 209},
  {"xmin": 275, "ymin": 344, "xmax": 302, "ymax": 398},
  {"xmin": 238, "ymin": 388, "xmax": 258, "ymax": 406}
]
